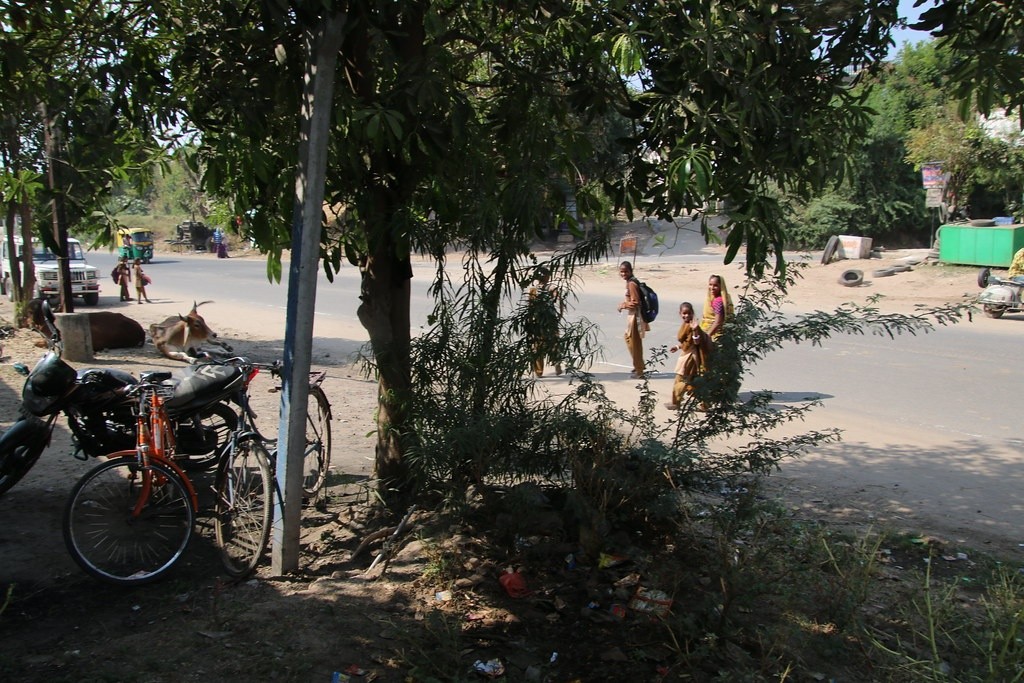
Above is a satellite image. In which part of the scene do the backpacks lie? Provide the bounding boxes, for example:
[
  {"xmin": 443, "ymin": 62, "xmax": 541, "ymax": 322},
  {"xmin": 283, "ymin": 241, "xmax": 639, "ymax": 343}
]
[
  {"xmin": 629, "ymin": 278, "xmax": 659, "ymax": 323},
  {"xmin": 112, "ymin": 266, "xmax": 120, "ymax": 284}
]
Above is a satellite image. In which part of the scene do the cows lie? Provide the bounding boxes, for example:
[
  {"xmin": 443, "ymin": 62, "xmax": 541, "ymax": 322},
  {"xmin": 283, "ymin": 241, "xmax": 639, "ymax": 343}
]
[{"xmin": 27, "ymin": 299, "xmax": 235, "ymax": 365}]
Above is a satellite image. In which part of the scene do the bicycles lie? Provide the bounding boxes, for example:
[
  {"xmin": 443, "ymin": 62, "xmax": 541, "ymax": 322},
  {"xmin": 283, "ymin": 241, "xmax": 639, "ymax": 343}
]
[
  {"xmin": 195, "ymin": 350, "xmax": 332, "ymax": 579},
  {"xmin": 62, "ymin": 370, "xmax": 198, "ymax": 586}
]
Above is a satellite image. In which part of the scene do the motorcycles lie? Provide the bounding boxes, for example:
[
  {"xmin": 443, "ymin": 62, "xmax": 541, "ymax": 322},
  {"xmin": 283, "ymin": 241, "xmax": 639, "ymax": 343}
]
[
  {"xmin": 978, "ymin": 268, "xmax": 1024, "ymax": 318},
  {"xmin": 0, "ymin": 300, "xmax": 259, "ymax": 493},
  {"xmin": 117, "ymin": 228, "xmax": 154, "ymax": 264}
]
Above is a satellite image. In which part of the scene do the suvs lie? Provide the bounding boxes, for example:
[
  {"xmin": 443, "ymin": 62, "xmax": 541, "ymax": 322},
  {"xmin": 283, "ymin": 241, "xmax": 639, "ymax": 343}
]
[{"xmin": 0, "ymin": 235, "xmax": 102, "ymax": 306}]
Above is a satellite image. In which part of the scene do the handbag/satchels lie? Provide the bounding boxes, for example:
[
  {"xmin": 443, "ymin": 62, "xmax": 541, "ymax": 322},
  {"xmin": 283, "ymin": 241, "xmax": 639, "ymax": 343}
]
[{"xmin": 141, "ymin": 275, "xmax": 151, "ymax": 285}]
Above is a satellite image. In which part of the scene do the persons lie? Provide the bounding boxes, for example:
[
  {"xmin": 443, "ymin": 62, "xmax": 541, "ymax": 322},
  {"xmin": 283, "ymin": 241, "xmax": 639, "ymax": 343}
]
[
  {"xmin": 617, "ymin": 261, "xmax": 650, "ymax": 379},
  {"xmin": 687, "ymin": 274, "xmax": 734, "ymax": 411},
  {"xmin": 132, "ymin": 257, "xmax": 152, "ymax": 304},
  {"xmin": 116, "ymin": 256, "xmax": 133, "ymax": 302},
  {"xmin": 218, "ymin": 237, "xmax": 229, "ymax": 258},
  {"xmin": 527, "ymin": 267, "xmax": 562, "ymax": 377},
  {"xmin": 663, "ymin": 301, "xmax": 710, "ymax": 411},
  {"xmin": 1008, "ymin": 247, "xmax": 1024, "ymax": 310}
]
